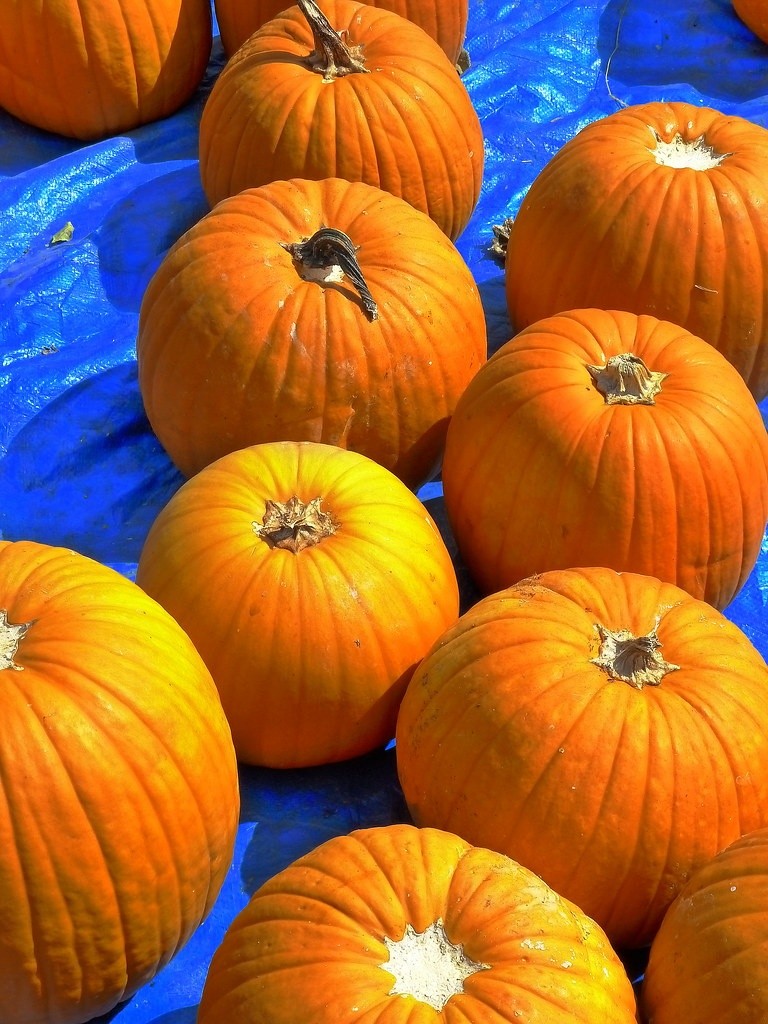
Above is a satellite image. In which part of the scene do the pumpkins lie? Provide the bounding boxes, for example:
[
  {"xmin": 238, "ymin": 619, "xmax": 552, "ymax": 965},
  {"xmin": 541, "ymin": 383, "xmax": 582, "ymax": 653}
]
[{"xmin": 0, "ymin": 0, "xmax": 768, "ymax": 1024}]
